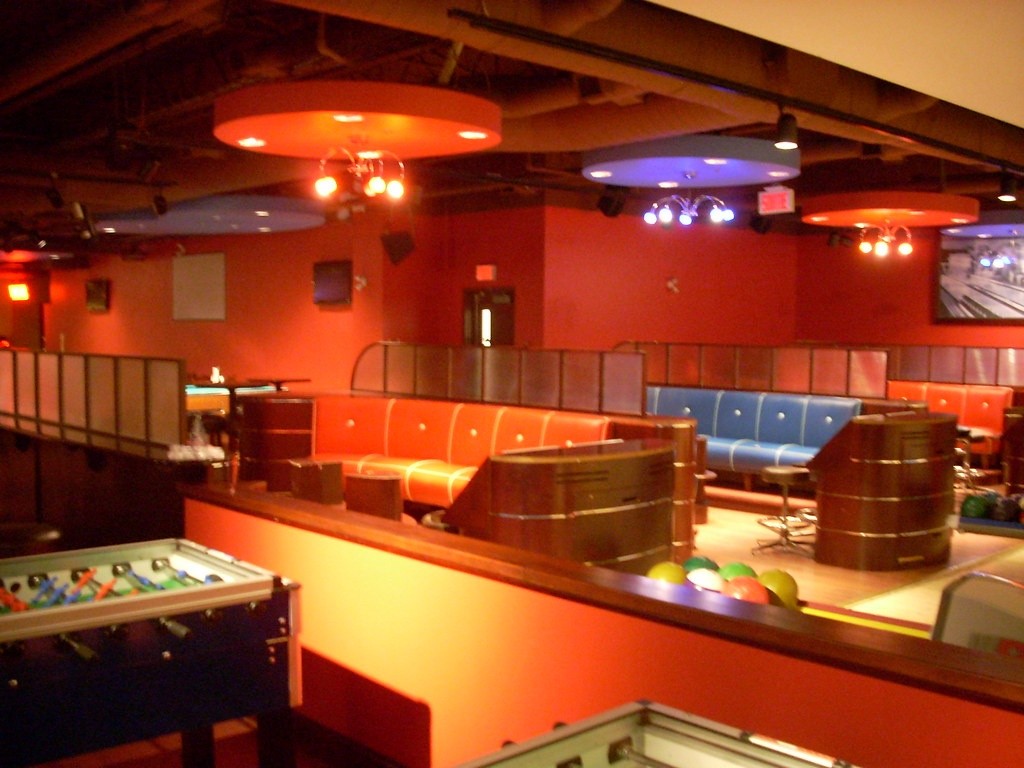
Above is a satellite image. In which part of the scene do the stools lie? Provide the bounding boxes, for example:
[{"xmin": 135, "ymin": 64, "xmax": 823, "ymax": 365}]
[
  {"xmin": 954, "ymin": 425, "xmax": 971, "ymax": 489},
  {"xmin": 748, "ymin": 466, "xmax": 814, "ymax": 559}
]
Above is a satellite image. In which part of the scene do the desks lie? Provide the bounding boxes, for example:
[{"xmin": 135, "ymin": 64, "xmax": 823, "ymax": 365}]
[{"xmin": 192, "ymin": 378, "xmax": 310, "ymax": 419}]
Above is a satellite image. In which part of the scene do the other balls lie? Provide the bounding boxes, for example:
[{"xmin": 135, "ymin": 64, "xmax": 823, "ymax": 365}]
[
  {"xmin": 644, "ymin": 556, "xmax": 800, "ymax": 608},
  {"xmin": 960, "ymin": 493, "xmax": 1024, "ymax": 525}
]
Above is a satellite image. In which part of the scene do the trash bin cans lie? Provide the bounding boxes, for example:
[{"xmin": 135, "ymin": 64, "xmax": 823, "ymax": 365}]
[{"xmin": 421, "ymin": 510, "xmax": 450, "ymax": 529}]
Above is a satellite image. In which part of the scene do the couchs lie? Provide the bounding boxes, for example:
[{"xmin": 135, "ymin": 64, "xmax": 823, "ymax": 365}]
[
  {"xmin": 642, "ymin": 383, "xmax": 862, "ymax": 493},
  {"xmin": 311, "ymin": 396, "xmax": 614, "ymax": 510},
  {"xmin": 883, "ymin": 378, "xmax": 1014, "ymax": 471}
]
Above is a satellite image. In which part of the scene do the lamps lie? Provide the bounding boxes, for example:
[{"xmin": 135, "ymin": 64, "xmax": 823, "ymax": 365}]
[
  {"xmin": 151, "ymin": 186, "xmax": 167, "ymax": 215},
  {"xmin": 45, "ymin": 176, "xmax": 62, "ymax": 209},
  {"xmin": 839, "ymin": 237, "xmax": 851, "ymax": 247},
  {"xmin": 774, "ymin": 104, "xmax": 799, "ymax": 150},
  {"xmin": 643, "ymin": 171, "xmax": 734, "ymax": 226},
  {"xmin": 998, "ymin": 164, "xmax": 1017, "ymax": 202},
  {"xmin": 825, "ymin": 232, "xmax": 840, "ymax": 247}
]
